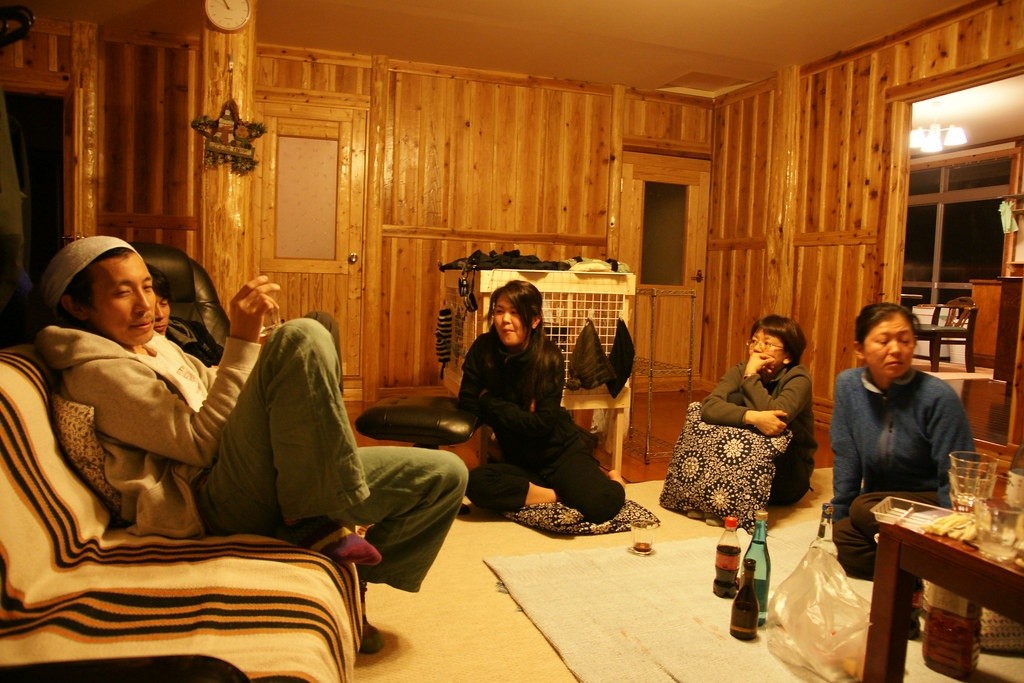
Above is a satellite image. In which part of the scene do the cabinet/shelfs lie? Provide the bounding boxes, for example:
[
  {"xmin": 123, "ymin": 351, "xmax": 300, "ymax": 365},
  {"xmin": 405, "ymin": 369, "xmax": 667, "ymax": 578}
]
[
  {"xmin": 969, "ymin": 278, "xmax": 1003, "ymax": 370},
  {"xmin": 630, "ymin": 288, "xmax": 698, "ymax": 465},
  {"xmin": 440, "ymin": 267, "xmax": 637, "ymax": 478},
  {"xmin": 998, "ymin": 194, "xmax": 1024, "ymax": 215},
  {"xmin": 992, "ymin": 275, "xmax": 1024, "ymax": 384}
]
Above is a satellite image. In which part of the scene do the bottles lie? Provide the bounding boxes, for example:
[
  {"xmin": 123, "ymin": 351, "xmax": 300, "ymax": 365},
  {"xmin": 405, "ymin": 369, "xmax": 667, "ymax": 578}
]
[
  {"xmin": 712, "ymin": 517, "xmax": 741, "ymax": 598},
  {"xmin": 1005, "ymin": 468, "xmax": 1024, "ymax": 508},
  {"xmin": 923, "ymin": 582, "xmax": 982, "ymax": 680},
  {"xmin": 908, "ymin": 577, "xmax": 924, "ymax": 639},
  {"xmin": 809, "ymin": 503, "xmax": 838, "ymax": 560},
  {"xmin": 730, "ymin": 558, "xmax": 759, "ymax": 640}
]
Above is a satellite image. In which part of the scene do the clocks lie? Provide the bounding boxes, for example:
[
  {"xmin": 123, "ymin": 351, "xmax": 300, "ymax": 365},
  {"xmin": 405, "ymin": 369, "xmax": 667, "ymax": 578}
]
[{"xmin": 204, "ymin": 0, "xmax": 252, "ymax": 33}]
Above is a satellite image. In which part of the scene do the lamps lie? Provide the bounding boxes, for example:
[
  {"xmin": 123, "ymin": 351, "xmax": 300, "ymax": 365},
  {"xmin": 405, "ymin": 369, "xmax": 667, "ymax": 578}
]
[{"xmin": 909, "ymin": 123, "xmax": 968, "ymax": 154}]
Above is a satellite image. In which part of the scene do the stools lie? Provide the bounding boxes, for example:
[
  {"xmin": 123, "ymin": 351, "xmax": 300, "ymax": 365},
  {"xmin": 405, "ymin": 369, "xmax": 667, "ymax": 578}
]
[{"xmin": 355, "ymin": 393, "xmax": 480, "ymax": 517}]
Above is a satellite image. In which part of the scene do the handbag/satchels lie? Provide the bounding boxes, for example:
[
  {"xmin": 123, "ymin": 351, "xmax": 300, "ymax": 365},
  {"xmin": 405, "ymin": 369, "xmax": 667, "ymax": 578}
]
[{"xmin": 764, "ymin": 547, "xmax": 870, "ymax": 681}]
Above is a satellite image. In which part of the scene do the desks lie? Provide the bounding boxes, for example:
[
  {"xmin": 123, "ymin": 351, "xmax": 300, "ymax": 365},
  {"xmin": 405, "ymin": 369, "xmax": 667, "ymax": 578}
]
[
  {"xmin": 901, "ymin": 293, "xmax": 924, "ymax": 299},
  {"xmin": 862, "ymin": 523, "xmax": 1024, "ymax": 683}
]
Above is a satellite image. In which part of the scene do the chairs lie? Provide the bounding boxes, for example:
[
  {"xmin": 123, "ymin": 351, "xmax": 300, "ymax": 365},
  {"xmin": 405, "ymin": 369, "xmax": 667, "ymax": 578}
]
[
  {"xmin": 912, "ymin": 296, "xmax": 978, "ymax": 373},
  {"xmin": 128, "ymin": 240, "xmax": 231, "ymax": 348}
]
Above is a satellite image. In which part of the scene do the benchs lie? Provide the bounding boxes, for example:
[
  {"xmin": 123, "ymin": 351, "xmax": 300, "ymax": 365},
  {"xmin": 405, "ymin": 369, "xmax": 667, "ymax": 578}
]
[{"xmin": 0, "ymin": 343, "xmax": 365, "ymax": 683}]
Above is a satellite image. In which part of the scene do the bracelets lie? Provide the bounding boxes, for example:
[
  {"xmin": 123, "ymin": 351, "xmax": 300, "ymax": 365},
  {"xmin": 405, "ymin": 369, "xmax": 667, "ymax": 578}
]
[{"xmin": 742, "ymin": 372, "xmax": 756, "ymax": 379}]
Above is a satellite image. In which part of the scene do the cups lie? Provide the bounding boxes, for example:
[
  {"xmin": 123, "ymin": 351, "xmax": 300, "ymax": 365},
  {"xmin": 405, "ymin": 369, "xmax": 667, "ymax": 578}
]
[
  {"xmin": 260, "ymin": 312, "xmax": 283, "ymax": 336},
  {"xmin": 976, "ymin": 500, "xmax": 1024, "ymax": 561},
  {"xmin": 1008, "ymin": 262, "xmax": 1024, "ymax": 276},
  {"xmin": 949, "ymin": 451, "xmax": 1000, "ymax": 480},
  {"xmin": 744, "ymin": 510, "xmax": 770, "ymax": 626},
  {"xmin": 947, "ymin": 470, "xmax": 997, "ymax": 513},
  {"xmin": 630, "ymin": 519, "xmax": 658, "ymax": 555}
]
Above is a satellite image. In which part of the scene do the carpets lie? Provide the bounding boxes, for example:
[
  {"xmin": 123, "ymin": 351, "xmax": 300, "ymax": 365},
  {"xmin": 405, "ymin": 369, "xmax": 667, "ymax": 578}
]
[
  {"xmin": 354, "ymin": 464, "xmax": 957, "ymax": 683},
  {"xmin": 485, "ymin": 519, "xmax": 1024, "ymax": 683},
  {"xmin": 482, "ymin": 518, "xmax": 1024, "ymax": 683}
]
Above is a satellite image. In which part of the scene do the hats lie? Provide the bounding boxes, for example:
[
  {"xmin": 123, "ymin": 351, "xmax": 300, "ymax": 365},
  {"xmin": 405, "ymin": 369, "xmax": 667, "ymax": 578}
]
[
  {"xmin": 40, "ymin": 236, "xmax": 143, "ymax": 314},
  {"xmin": 567, "ymin": 320, "xmax": 617, "ymax": 390}
]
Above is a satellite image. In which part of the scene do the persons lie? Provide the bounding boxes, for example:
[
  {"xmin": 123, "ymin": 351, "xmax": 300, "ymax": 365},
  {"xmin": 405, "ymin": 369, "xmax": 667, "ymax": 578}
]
[
  {"xmin": 829, "ymin": 303, "xmax": 977, "ymax": 640},
  {"xmin": 34, "ymin": 237, "xmax": 469, "ymax": 655},
  {"xmin": 458, "ymin": 280, "xmax": 626, "ymax": 525},
  {"xmin": 687, "ymin": 312, "xmax": 818, "ymax": 528}
]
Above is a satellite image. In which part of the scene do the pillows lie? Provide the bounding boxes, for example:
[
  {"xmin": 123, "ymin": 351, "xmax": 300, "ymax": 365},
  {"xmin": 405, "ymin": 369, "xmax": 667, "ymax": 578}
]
[
  {"xmin": 500, "ymin": 496, "xmax": 660, "ymax": 536},
  {"xmin": 51, "ymin": 392, "xmax": 120, "ymax": 527},
  {"xmin": 660, "ymin": 402, "xmax": 794, "ymax": 537}
]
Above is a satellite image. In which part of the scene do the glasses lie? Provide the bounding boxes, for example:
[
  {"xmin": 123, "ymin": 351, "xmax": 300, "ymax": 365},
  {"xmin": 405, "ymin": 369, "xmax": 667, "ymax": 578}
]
[{"xmin": 747, "ymin": 340, "xmax": 785, "ymax": 349}]
